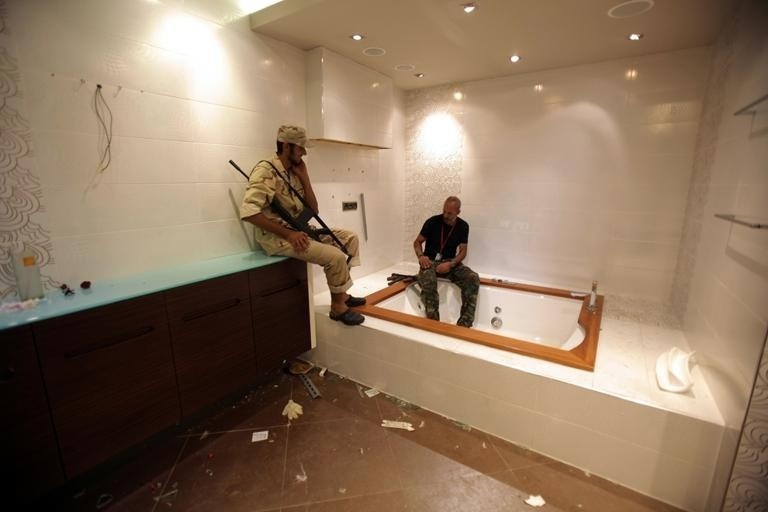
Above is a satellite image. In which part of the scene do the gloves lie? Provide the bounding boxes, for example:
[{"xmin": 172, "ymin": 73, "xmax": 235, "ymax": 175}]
[{"xmin": 281, "ymin": 399, "xmax": 304, "ymax": 421}]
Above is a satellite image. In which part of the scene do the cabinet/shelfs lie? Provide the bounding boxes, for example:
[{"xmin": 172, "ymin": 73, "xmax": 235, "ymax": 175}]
[
  {"xmin": 32, "ymin": 292, "xmax": 182, "ymax": 479},
  {"xmin": 306, "ymin": 47, "xmax": 392, "ymax": 151},
  {"xmin": 249, "ymin": 258, "xmax": 312, "ymax": 380},
  {"xmin": 0, "ymin": 324, "xmax": 66, "ymax": 511},
  {"xmin": 164, "ymin": 270, "xmax": 259, "ymax": 424}
]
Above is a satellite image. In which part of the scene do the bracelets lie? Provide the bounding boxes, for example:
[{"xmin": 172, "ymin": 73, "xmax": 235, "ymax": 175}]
[
  {"xmin": 417, "ymin": 254, "xmax": 423, "ymax": 258},
  {"xmin": 447, "ymin": 260, "xmax": 455, "ymax": 268}
]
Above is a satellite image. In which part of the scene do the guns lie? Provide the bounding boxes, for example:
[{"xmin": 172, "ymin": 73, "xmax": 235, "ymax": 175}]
[
  {"xmin": 387, "ymin": 273, "xmax": 417, "ymax": 285},
  {"xmin": 228, "ymin": 159, "xmax": 350, "ymax": 264}
]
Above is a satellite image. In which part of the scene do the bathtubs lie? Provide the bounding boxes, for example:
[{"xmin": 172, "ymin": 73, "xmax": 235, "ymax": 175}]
[{"xmin": 370, "ymin": 278, "xmax": 588, "ymax": 352}]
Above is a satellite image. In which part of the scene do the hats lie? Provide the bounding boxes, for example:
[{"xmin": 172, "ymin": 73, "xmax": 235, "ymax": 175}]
[{"xmin": 276, "ymin": 125, "xmax": 316, "ymax": 148}]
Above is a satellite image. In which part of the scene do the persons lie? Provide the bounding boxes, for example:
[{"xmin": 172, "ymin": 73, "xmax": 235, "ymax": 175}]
[
  {"xmin": 412, "ymin": 194, "xmax": 480, "ymax": 328},
  {"xmin": 239, "ymin": 124, "xmax": 367, "ymax": 325}
]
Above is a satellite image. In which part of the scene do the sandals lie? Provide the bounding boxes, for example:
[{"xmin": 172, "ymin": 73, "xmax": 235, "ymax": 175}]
[{"xmin": 329, "ymin": 294, "xmax": 366, "ymax": 326}]
